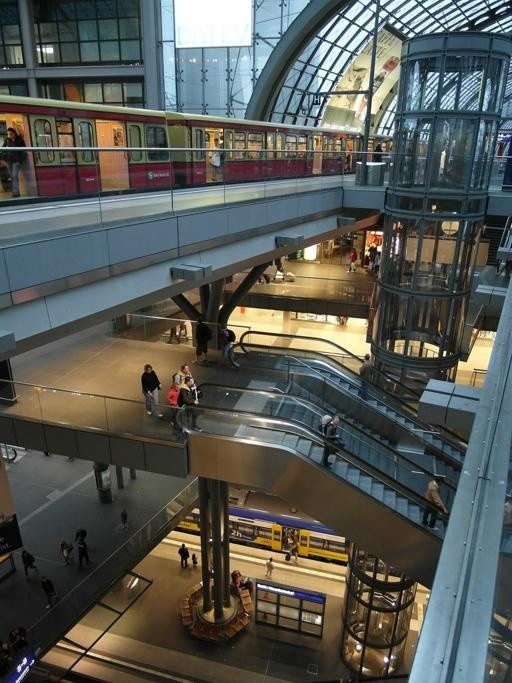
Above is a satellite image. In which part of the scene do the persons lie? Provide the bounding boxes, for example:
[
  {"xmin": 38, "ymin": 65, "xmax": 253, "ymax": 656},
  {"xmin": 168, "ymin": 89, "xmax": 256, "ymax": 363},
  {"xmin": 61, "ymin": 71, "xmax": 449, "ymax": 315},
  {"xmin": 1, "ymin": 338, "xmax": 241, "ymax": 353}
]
[
  {"xmin": 498, "ymin": 495, "xmax": 512, "ymax": 549},
  {"xmin": 73, "ymin": 526, "xmax": 88, "ymax": 542},
  {"xmin": 209, "ymin": 565, "xmax": 215, "ymax": 579},
  {"xmin": 178, "ymin": 543, "xmax": 190, "ymax": 568},
  {"xmin": 1, "ymin": 638, "xmax": 16, "ymax": 671},
  {"xmin": 192, "ymin": 553, "xmax": 198, "ymax": 566},
  {"xmin": 220, "ymin": 323, "xmax": 236, "ymax": 365},
  {"xmin": 265, "ymin": 557, "xmax": 274, "ymax": 577},
  {"xmin": 289, "ymin": 539, "xmax": 298, "ymax": 561},
  {"xmin": 179, "ymin": 375, "xmax": 204, "ymax": 431},
  {"xmin": 165, "ymin": 381, "xmax": 182, "ymax": 430},
  {"xmin": 321, "ymin": 414, "xmax": 340, "ymax": 471},
  {"xmin": 172, "ymin": 363, "xmax": 194, "ymax": 390},
  {"xmin": 367, "ymin": 245, "xmax": 381, "ymax": 279},
  {"xmin": 120, "ymin": 508, "xmax": 128, "ymax": 530},
  {"xmin": 231, "ymin": 569, "xmax": 240, "ymax": 589},
  {"xmin": 21, "ymin": 549, "xmax": 39, "ymax": 582},
  {"xmin": 208, "ymin": 137, "xmax": 221, "ymax": 180},
  {"xmin": 8, "ymin": 624, "xmax": 29, "ymax": 649},
  {"xmin": 373, "ymin": 143, "xmax": 382, "ymax": 162},
  {"xmin": 178, "ymin": 322, "xmax": 189, "ymax": 342},
  {"xmin": 422, "ymin": 475, "xmax": 449, "ymax": 530},
  {"xmin": 141, "ymin": 363, "xmax": 164, "ymax": 418},
  {"xmin": 57, "ymin": 536, "xmax": 75, "ymax": 567},
  {"xmin": 166, "ymin": 326, "xmax": 181, "ymax": 343},
  {"xmin": 239, "ymin": 575, "xmax": 254, "ymax": 595},
  {"xmin": 346, "ymin": 246, "xmax": 357, "ymax": 272},
  {"xmin": 39, "ymin": 575, "xmax": 61, "ymax": 608},
  {"xmin": 357, "ymin": 351, "xmax": 374, "ymax": 400},
  {"xmin": 191, "ymin": 316, "xmax": 209, "ymax": 364},
  {"xmin": 1, "ymin": 125, "xmax": 28, "ymax": 197},
  {"xmin": 77, "ymin": 536, "xmax": 90, "ymax": 568}
]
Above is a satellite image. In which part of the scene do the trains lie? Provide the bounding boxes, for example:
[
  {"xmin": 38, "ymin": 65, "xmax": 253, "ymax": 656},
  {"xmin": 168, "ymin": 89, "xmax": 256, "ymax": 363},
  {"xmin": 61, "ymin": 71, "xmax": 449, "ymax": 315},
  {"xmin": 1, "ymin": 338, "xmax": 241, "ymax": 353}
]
[{"xmin": 0, "ymin": 95, "xmax": 429, "ymax": 207}]
